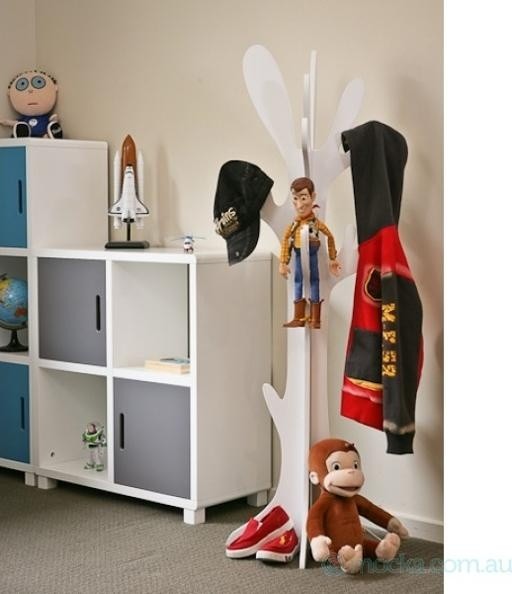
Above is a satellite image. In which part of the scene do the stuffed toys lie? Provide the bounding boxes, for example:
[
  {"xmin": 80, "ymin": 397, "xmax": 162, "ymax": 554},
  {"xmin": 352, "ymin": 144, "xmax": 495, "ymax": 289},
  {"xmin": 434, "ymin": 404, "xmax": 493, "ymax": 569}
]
[{"xmin": 302, "ymin": 439, "xmax": 408, "ymax": 576}]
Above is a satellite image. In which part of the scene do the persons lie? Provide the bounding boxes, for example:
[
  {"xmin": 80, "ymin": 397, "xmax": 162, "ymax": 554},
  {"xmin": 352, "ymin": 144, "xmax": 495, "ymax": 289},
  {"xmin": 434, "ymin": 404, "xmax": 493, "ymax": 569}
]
[
  {"xmin": 0, "ymin": 68, "xmax": 65, "ymax": 138},
  {"xmin": 278, "ymin": 175, "xmax": 341, "ymax": 329},
  {"xmin": 79, "ymin": 419, "xmax": 106, "ymax": 473}
]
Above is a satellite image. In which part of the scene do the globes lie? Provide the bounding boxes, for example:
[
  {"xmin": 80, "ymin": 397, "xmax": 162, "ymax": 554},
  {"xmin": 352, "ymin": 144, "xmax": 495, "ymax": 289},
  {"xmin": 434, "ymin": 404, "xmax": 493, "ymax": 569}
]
[{"xmin": 0, "ymin": 273, "xmax": 28, "ymax": 352}]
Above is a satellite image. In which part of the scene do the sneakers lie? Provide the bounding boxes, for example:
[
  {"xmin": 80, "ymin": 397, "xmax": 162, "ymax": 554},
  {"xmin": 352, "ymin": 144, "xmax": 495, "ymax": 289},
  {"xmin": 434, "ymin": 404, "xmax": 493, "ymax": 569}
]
[
  {"xmin": 256, "ymin": 526, "xmax": 301, "ymax": 564},
  {"xmin": 225, "ymin": 504, "xmax": 295, "ymax": 559}
]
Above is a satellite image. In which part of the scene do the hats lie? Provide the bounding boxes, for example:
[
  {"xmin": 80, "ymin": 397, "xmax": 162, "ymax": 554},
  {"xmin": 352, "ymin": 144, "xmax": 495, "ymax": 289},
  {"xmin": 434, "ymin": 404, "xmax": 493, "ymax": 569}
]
[{"xmin": 214, "ymin": 160, "xmax": 275, "ymax": 263}]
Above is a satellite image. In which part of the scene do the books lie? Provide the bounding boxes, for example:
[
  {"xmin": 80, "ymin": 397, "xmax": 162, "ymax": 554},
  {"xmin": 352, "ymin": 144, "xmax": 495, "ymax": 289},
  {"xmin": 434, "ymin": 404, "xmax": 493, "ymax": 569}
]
[{"xmin": 144, "ymin": 354, "xmax": 190, "ymax": 375}]
